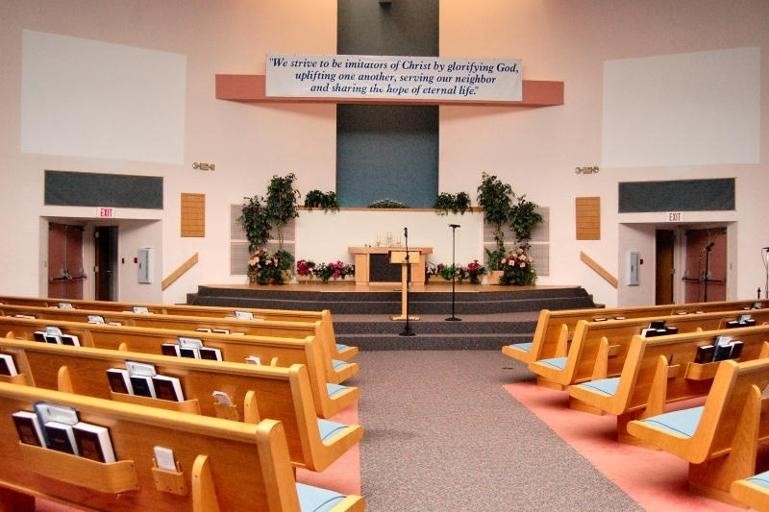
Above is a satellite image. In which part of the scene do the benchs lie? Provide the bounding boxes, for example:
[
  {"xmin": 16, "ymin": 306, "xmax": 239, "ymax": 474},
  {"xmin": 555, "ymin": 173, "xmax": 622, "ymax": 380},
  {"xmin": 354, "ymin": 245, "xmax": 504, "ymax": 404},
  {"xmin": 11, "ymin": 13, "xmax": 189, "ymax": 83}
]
[
  {"xmin": 1, "ymin": 292, "xmax": 369, "ymax": 511},
  {"xmin": 498, "ymin": 297, "xmax": 769, "ymax": 512}
]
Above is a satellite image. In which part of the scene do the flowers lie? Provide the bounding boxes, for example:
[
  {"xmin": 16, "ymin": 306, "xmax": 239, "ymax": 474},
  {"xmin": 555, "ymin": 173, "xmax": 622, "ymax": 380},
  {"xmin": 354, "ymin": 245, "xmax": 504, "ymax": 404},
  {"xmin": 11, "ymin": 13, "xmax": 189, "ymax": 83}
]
[
  {"xmin": 367, "ymin": 196, "xmax": 409, "ymax": 209},
  {"xmin": 424, "ymin": 239, "xmax": 538, "ymax": 288},
  {"xmin": 246, "ymin": 244, "xmax": 355, "ymax": 287}
]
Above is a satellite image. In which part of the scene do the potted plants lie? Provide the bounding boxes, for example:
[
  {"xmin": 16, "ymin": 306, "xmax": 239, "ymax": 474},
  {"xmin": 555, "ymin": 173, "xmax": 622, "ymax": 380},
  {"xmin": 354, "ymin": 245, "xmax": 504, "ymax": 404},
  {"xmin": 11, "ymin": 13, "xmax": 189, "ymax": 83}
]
[{"xmin": 301, "ymin": 187, "xmax": 341, "ymax": 214}]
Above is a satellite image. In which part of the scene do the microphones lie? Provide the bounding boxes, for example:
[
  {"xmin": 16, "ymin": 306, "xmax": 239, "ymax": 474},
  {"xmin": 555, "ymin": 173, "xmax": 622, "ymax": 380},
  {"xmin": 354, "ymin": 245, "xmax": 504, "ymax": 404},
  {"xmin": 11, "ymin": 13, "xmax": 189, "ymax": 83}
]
[
  {"xmin": 705, "ymin": 242, "xmax": 713, "ymax": 249},
  {"xmin": 404, "ymin": 227, "xmax": 407, "ymax": 235},
  {"xmin": 449, "ymin": 224, "xmax": 459, "ymax": 227}
]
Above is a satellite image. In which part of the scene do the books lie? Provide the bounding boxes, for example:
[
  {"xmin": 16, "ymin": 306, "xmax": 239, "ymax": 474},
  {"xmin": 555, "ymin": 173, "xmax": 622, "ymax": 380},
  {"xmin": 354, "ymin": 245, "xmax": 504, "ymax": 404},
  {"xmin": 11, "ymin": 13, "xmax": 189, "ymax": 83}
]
[
  {"xmin": 28, "ymin": 300, "xmax": 263, "ymax": 361},
  {"xmin": 0, "ymin": 353, "xmax": 18, "ymax": 377},
  {"xmin": 725, "ymin": 318, "xmax": 756, "ymax": 329},
  {"xmin": 641, "ymin": 325, "xmax": 678, "ymax": 338},
  {"xmin": 105, "ymin": 367, "xmax": 186, "ymax": 403},
  {"xmin": 694, "ymin": 340, "xmax": 744, "ymax": 364},
  {"xmin": 11, "ymin": 409, "xmax": 117, "ymax": 463}
]
